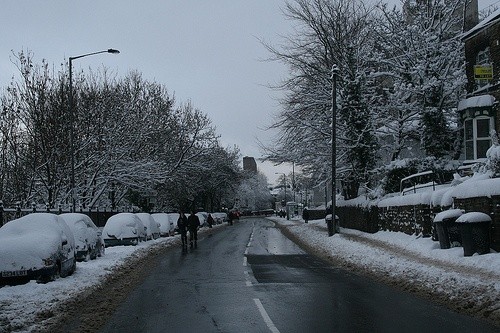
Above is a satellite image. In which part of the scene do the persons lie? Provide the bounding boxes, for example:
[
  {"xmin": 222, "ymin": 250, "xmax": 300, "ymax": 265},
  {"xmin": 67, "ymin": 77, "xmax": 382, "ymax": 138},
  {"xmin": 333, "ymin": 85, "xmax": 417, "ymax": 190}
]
[
  {"xmin": 177, "ymin": 211, "xmax": 188, "ymax": 248},
  {"xmin": 276, "ymin": 210, "xmax": 286, "ymax": 218},
  {"xmin": 303, "ymin": 208, "xmax": 309, "ymax": 223},
  {"xmin": 207, "ymin": 214, "xmax": 213, "ymax": 228},
  {"xmin": 187, "ymin": 209, "xmax": 200, "ymax": 248}
]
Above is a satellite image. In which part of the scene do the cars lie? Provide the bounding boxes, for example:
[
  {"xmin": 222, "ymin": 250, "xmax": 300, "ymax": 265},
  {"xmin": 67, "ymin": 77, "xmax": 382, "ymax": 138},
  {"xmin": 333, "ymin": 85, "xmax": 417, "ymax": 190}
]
[
  {"xmin": 101, "ymin": 213, "xmax": 149, "ymax": 247},
  {"xmin": 151, "ymin": 212, "xmax": 175, "ymax": 237},
  {"xmin": 136, "ymin": 212, "xmax": 160, "ymax": 240},
  {"xmin": 169, "ymin": 213, "xmax": 180, "ymax": 234},
  {"xmin": 58, "ymin": 213, "xmax": 106, "ymax": 262},
  {"xmin": 0, "ymin": 213, "xmax": 77, "ymax": 289},
  {"xmin": 184, "ymin": 212, "xmax": 227, "ymax": 231}
]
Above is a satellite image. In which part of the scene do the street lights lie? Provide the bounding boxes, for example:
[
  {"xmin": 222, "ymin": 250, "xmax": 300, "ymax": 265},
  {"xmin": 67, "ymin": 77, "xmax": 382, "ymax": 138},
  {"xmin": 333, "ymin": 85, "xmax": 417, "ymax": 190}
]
[
  {"xmin": 275, "ymin": 172, "xmax": 286, "ymax": 204},
  {"xmin": 69, "ymin": 48, "xmax": 120, "ymax": 211}
]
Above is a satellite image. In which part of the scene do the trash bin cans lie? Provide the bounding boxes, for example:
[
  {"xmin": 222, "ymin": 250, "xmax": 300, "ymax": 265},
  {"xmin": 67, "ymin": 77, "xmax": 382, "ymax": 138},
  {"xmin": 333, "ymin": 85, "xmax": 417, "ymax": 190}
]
[
  {"xmin": 454, "ymin": 219, "xmax": 492, "ymax": 256},
  {"xmin": 326, "ymin": 218, "xmax": 340, "ymax": 236},
  {"xmin": 433, "ymin": 216, "xmax": 460, "ymax": 249}
]
